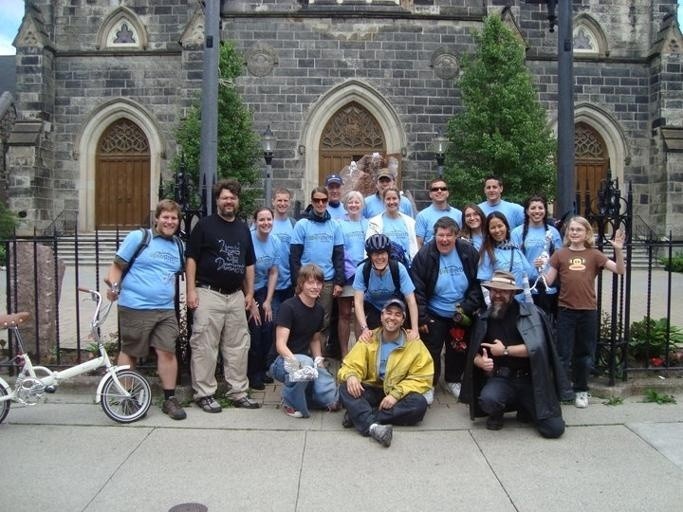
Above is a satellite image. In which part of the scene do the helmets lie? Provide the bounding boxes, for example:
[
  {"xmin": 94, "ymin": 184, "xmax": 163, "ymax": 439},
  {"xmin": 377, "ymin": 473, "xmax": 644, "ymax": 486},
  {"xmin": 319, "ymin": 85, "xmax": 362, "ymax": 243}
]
[{"xmin": 366, "ymin": 233, "xmax": 391, "ymax": 256}]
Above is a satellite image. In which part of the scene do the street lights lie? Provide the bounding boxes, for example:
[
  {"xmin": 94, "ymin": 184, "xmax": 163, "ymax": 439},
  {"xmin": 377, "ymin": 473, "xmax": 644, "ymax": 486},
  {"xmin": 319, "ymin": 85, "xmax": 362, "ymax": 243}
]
[
  {"xmin": 432, "ymin": 129, "xmax": 450, "ymax": 178},
  {"xmin": 260, "ymin": 124, "xmax": 276, "ymax": 208}
]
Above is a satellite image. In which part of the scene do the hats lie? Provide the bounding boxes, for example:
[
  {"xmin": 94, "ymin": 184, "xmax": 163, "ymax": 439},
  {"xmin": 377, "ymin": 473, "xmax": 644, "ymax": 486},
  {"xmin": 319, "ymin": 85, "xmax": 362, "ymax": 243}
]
[
  {"xmin": 327, "ymin": 175, "xmax": 344, "ymax": 187},
  {"xmin": 383, "ymin": 299, "xmax": 406, "ymax": 313},
  {"xmin": 377, "ymin": 170, "xmax": 394, "ymax": 182},
  {"xmin": 480, "ymin": 270, "xmax": 524, "ymax": 296}
]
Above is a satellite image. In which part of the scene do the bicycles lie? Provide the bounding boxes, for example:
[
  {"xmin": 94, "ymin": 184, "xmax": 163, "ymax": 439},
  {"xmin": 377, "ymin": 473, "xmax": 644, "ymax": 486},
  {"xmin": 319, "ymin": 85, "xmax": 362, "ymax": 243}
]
[{"xmin": 0, "ymin": 278, "xmax": 153, "ymax": 426}]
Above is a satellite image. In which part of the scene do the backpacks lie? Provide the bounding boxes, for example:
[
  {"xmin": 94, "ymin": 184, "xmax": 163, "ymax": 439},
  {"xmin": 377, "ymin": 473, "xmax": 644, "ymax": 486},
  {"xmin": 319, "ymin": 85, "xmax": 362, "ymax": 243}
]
[{"xmin": 364, "ymin": 238, "xmax": 409, "ymax": 289}]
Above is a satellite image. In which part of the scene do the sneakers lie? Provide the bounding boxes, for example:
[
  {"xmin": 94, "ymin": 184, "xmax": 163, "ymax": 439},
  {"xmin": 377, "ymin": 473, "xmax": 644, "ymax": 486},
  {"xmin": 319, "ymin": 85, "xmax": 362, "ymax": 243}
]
[
  {"xmin": 424, "ymin": 386, "xmax": 434, "ymax": 405},
  {"xmin": 196, "ymin": 396, "xmax": 221, "ymax": 413},
  {"xmin": 371, "ymin": 423, "xmax": 393, "ymax": 447},
  {"xmin": 161, "ymin": 397, "xmax": 185, "ymax": 420},
  {"xmin": 343, "ymin": 410, "xmax": 352, "ymax": 427},
  {"xmin": 235, "ymin": 396, "xmax": 261, "ymax": 409},
  {"xmin": 281, "ymin": 402, "xmax": 303, "ymax": 418},
  {"xmin": 448, "ymin": 382, "xmax": 461, "ymax": 396},
  {"xmin": 123, "ymin": 399, "xmax": 146, "ymax": 420},
  {"xmin": 262, "ymin": 374, "xmax": 273, "ymax": 382},
  {"xmin": 252, "ymin": 381, "xmax": 264, "ymax": 389},
  {"xmin": 489, "ymin": 412, "xmax": 503, "ymax": 429},
  {"xmin": 575, "ymin": 391, "xmax": 589, "ymax": 408}
]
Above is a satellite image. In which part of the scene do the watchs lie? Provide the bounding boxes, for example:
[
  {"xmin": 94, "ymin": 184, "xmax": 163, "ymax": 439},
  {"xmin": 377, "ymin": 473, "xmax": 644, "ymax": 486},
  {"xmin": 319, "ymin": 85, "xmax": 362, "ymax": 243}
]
[
  {"xmin": 360, "ymin": 325, "xmax": 368, "ymax": 330},
  {"xmin": 503, "ymin": 345, "xmax": 509, "ymax": 357}
]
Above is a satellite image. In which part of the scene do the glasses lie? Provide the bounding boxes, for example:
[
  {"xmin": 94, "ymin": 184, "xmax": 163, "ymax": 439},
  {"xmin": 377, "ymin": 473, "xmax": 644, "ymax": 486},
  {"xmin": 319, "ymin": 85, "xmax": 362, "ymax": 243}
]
[
  {"xmin": 219, "ymin": 196, "xmax": 238, "ymax": 200},
  {"xmin": 312, "ymin": 198, "xmax": 328, "ymax": 204},
  {"xmin": 430, "ymin": 187, "xmax": 447, "ymax": 191}
]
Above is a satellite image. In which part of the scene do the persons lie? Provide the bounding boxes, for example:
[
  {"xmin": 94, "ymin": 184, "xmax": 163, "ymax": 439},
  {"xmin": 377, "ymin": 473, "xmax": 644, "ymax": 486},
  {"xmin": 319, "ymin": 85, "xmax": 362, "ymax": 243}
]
[
  {"xmin": 188, "ymin": 179, "xmax": 261, "ymax": 413},
  {"xmin": 336, "ymin": 189, "xmax": 367, "ymax": 357},
  {"xmin": 268, "ymin": 184, "xmax": 297, "ymax": 295},
  {"xmin": 460, "ymin": 204, "xmax": 485, "ymax": 249},
  {"xmin": 362, "ymin": 168, "xmax": 413, "ymax": 219},
  {"xmin": 287, "ymin": 186, "xmax": 345, "ymax": 348},
  {"xmin": 268, "ymin": 264, "xmax": 340, "ymax": 419},
  {"xmin": 535, "ymin": 216, "xmax": 626, "ymax": 411},
  {"xmin": 324, "ymin": 174, "xmax": 346, "ymax": 221},
  {"xmin": 244, "ymin": 204, "xmax": 279, "ymax": 389},
  {"xmin": 408, "ymin": 217, "xmax": 483, "ymax": 404},
  {"xmin": 353, "ymin": 235, "xmax": 421, "ymax": 343},
  {"xmin": 335, "ymin": 298, "xmax": 434, "ymax": 447},
  {"xmin": 415, "ymin": 176, "xmax": 464, "ymax": 247},
  {"xmin": 365, "ymin": 188, "xmax": 419, "ymax": 263},
  {"xmin": 106, "ymin": 200, "xmax": 187, "ymax": 421},
  {"xmin": 458, "ymin": 271, "xmax": 571, "ymax": 442},
  {"xmin": 510, "ymin": 195, "xmax": 563, "ymax": 310},
  {"xmin": 477, "ymin": 211, "xmax": 534, "ymax": 308},
  {"xmin": 477, "ymin": 177, "xmax": 525, "ymax": 231}
]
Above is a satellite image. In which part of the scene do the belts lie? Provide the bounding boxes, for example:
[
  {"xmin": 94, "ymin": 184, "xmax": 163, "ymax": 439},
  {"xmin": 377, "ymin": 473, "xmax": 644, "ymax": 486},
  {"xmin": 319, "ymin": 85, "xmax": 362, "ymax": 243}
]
[{"xmin": 195, "ymin": 282, "xmax": 242, "ymax": 294}]
